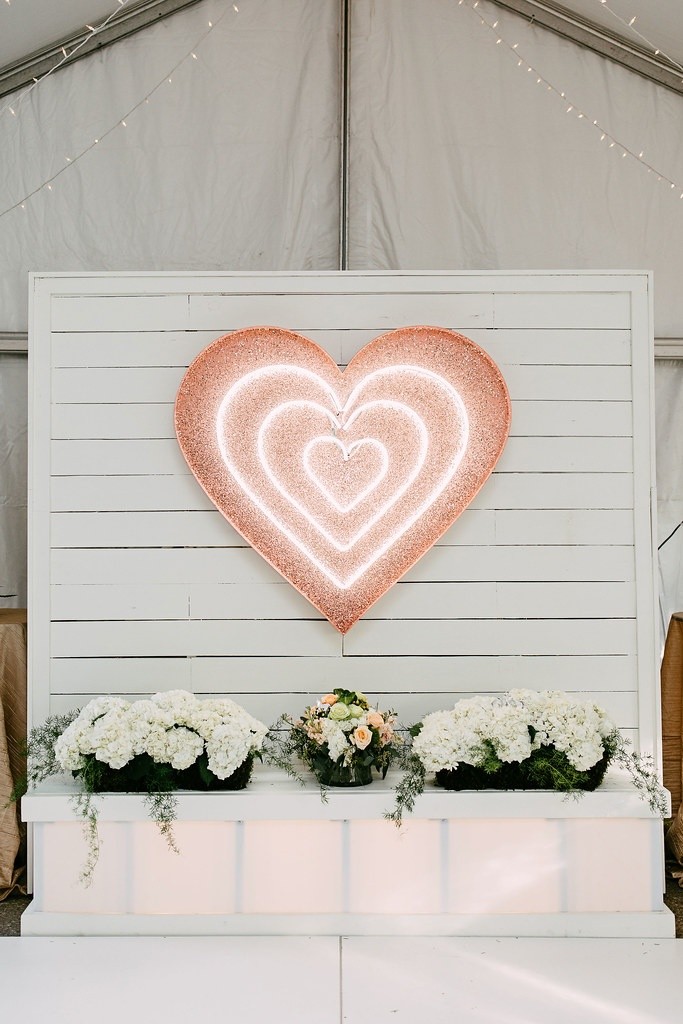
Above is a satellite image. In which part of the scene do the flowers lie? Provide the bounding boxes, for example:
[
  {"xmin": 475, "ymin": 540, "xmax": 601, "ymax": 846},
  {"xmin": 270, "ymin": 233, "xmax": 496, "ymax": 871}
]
[
  {"xmin": 380, "ymin": 688, "xmax": 671, "ymax": 828},
  {"xmin": 291, "ymin": 688, "xmax": 405, "ymax": 779},
  {"xmin": 2, "ymin": 689, "xmax": 331, "ymax": 891}
]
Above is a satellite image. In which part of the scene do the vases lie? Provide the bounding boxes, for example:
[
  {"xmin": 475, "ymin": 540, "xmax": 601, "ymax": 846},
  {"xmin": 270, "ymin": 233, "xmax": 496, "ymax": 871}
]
[
  {"xmin": 80, "ymin": 753, "xmax": 254, "ymax": 792},
  {"xmin": 315, "ymin": 754, "xmax": 373, "ymax": 788},
  {"xmin": 434, "ymin": 743, "xmax": 607, "ymax": 792}
]
